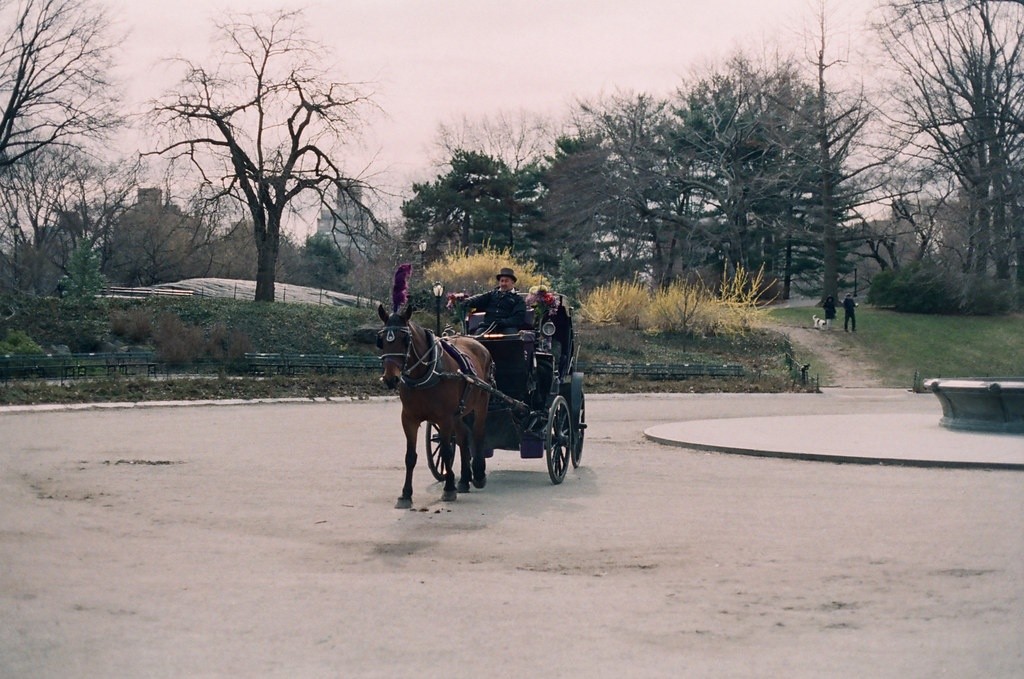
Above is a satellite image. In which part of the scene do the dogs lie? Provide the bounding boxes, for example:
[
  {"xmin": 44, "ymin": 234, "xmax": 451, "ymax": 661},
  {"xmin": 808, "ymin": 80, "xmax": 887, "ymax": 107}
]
[{"xmin": 813, "ymin": 315, "xmax": 827, "ymax": 333}]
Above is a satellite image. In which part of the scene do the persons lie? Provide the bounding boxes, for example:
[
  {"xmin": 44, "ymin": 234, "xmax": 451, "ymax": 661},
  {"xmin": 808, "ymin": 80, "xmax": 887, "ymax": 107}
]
[
  {"xmin": 462, "ymin": 268, "xmax": 526, "ymax": 339},
  {"xmin": 843, "ymin": 294, "xmax": 858, "ymax": 331},
  {"xmin": 823, "ymin": 295, "xmax": 836, "ymax": 330}
]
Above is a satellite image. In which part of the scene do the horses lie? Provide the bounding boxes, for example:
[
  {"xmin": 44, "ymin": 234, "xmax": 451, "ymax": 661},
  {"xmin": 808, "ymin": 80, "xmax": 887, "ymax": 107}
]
[{"xmin": 375, "ymin": 303, "xmax": 496, "ymax": 510}]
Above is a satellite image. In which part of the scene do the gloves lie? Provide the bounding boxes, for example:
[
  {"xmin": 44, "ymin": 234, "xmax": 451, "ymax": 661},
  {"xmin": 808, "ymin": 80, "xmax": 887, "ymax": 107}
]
[{"xmin": 495, "ymin": 318, "xmax": 506, "ymax": 328}]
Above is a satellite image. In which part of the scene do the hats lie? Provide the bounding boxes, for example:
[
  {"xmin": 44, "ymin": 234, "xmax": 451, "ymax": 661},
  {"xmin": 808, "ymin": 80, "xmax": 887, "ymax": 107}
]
[{"xmin": 496, "ymin": 268, "xmax": 517, "ymax": 282}]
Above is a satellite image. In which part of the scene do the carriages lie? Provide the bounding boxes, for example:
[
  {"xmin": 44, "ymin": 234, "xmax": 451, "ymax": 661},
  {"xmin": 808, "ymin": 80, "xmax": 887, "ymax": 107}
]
[{"xmin": 378, "ymin": 265, "xmax": 587, "ymax": 511}]
